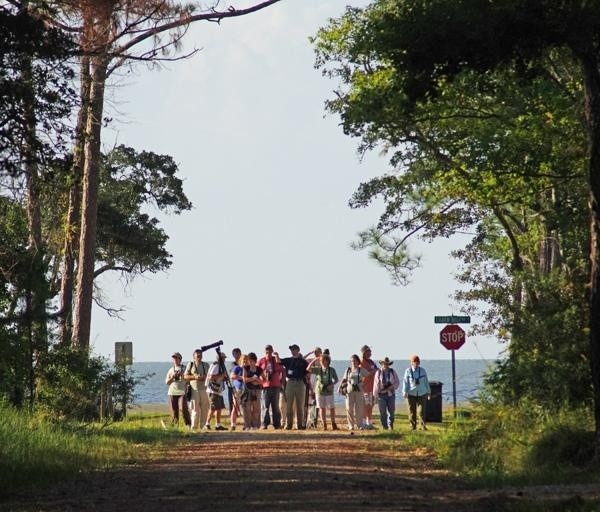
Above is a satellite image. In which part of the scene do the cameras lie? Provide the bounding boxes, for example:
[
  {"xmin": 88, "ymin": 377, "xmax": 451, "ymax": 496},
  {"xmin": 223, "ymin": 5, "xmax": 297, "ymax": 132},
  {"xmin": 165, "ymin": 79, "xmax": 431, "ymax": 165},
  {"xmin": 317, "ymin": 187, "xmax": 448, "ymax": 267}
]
[
  {"xmin": 352, "ymin": 384, "xmax": 360, "ymax": 391},
  {"xmin": 413, "ymin": 377, "xmax": 419, "ymax": 385},
  {"xmin": 266, "ymin": 373, "xmax": 271, "ymax": 380},
  {"xmin": 173, "ymin": 373, "xmax": 181, "ymax": 380},
  {"xmin": 201, "ymin": 340, "xmax": 223, "ymax": 352},
  {"xmin": 384, "ymin": 381, "xmax": 391, "ymax": 389}
]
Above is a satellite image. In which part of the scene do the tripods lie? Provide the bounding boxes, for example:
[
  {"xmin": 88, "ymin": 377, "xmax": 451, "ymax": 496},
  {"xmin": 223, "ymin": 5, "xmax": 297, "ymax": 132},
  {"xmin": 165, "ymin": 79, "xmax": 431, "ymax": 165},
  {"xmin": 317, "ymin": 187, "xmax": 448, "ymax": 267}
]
[{"xmin": 215, "ymin": 348, "xmax": 242, "ymax": 416}]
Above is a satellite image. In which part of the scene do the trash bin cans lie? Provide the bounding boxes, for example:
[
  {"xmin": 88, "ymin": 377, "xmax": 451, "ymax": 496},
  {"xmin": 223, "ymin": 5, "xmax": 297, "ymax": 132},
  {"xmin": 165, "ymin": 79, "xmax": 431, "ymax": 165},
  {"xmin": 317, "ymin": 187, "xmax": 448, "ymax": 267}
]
[{"xmin": 422, "ymin": 382, "xmax": 444, "ymax": 422}]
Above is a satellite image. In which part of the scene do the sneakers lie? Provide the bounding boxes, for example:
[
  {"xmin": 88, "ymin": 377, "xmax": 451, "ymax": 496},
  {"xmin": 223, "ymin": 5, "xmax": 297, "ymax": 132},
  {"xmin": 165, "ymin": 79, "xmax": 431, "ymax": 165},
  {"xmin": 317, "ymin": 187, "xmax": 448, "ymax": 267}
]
[{"xmin": 203, "ymin": 423, "xmax": 376, "ymax": 430}]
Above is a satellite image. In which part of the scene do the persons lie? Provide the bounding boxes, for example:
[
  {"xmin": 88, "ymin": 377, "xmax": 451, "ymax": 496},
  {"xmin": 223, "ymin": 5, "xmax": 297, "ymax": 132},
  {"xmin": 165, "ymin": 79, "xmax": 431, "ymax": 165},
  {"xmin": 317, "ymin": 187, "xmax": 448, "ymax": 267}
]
[
  {"xmin": 165, "ymin": 344, "xmax": 400, "ymax": 431},
  {"xmin": 402, "ymin": 355, "xmax": 432, "ymax": 431}
]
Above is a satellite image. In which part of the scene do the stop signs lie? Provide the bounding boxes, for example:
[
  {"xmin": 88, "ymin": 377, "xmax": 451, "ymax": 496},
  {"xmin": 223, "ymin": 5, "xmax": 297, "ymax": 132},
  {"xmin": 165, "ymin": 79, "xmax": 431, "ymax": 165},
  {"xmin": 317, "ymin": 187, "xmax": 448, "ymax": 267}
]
[{"xmin": 440, "ymin": 326, "xmax": 466, "ymax": 350}]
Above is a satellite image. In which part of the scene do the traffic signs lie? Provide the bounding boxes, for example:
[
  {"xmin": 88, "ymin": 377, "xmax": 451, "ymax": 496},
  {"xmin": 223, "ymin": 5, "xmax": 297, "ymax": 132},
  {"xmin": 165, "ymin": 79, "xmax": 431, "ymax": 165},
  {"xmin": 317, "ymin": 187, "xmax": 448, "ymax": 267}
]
[{"xmin": 435, "ymin": 316, "xmax": 471, "ymax": 324}]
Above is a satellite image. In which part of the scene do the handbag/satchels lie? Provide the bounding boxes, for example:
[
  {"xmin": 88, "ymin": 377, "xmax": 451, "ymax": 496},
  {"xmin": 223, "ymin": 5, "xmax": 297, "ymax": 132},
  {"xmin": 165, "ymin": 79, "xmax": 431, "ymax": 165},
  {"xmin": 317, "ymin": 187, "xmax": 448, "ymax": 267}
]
[
  {"xmin": 184, "ymin": 382, "xmax": 191, "ymax": 401},
  {"xmin": 339, "ymin": 379, "xmax": 347, "ymax": 395},
  {"xmin": 209, "ymin": 377, "xmax": 220, "ymax": 392},
  {"xmin": 347, "ymin": 381, "xmax": 364, "ymax": 395}
]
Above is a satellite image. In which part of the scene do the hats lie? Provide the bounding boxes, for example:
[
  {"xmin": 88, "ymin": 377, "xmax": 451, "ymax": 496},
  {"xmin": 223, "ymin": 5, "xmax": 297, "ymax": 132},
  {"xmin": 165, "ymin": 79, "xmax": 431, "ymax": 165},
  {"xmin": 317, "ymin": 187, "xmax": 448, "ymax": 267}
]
[
  {"xmin": 361, "ymin": 345, "xmax": 419, "ymax": 365},
  {"xmin": 171, "ymin": 344, "xmax": 300, "ymax": 360}
]
